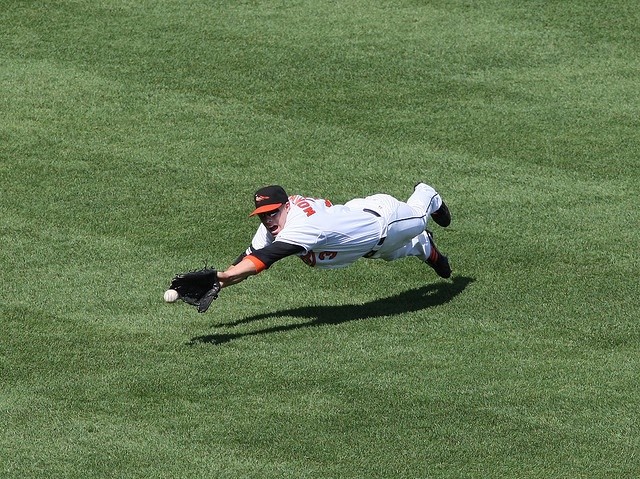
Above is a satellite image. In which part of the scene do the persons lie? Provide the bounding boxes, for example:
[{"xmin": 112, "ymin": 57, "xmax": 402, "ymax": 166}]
[{"xmin": 169, "ymin": 180, "xmax": 452, "ymax": 314}]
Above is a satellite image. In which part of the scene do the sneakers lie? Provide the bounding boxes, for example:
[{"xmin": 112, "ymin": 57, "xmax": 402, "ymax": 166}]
[
  {"xmin": 414, "ymin": 182, "xmax": 451, "ymax": 227},
  {"xmin": 425, "ymin": 230, "xmax": 450, "ymax": 278}
]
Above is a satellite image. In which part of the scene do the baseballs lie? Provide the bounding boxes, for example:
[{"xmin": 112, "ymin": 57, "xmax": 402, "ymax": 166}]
[{"xmin": 163, "ymin": 288, "xmax": 179, "ymax": 303}]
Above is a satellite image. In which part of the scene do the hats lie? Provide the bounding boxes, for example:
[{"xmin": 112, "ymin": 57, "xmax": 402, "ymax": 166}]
[{"xmin": 250, "ymin": 185, "xmax": 288, "ymax": 217}]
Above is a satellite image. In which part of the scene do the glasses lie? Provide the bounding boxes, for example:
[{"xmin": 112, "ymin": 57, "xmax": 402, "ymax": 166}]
[{"xmin": 257, "ymin": 206, "xmax": 283, "ymax": 218}]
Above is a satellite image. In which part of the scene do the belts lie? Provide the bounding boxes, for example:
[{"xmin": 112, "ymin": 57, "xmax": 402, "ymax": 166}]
[{"xmin": 363, "ymin": 206, "xmax": 385, "ymax": 258}]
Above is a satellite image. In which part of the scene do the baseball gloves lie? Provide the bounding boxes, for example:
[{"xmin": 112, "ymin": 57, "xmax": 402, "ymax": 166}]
[{"xmin": 168, "ymin": 268, "xmax": 221, "ymax": 313}]
